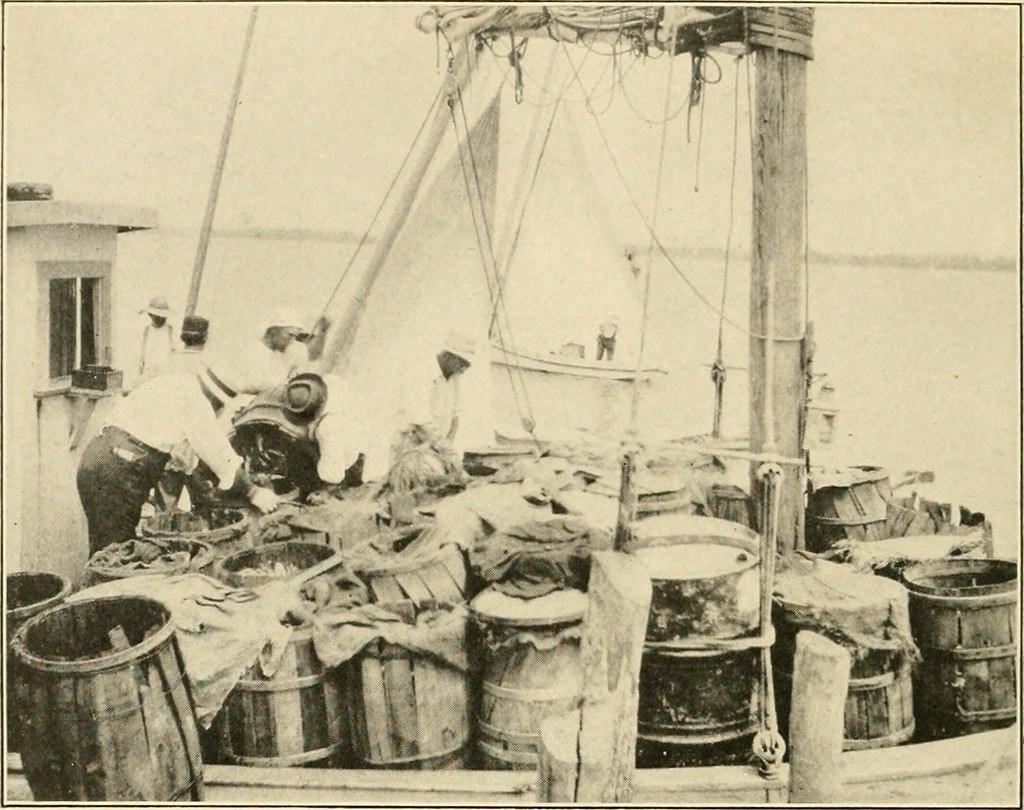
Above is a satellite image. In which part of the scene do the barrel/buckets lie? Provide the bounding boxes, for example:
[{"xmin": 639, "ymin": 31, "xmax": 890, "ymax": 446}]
[{"xmin": 8, "ymin": 389, "xmax": 1016, "ymax": 801}]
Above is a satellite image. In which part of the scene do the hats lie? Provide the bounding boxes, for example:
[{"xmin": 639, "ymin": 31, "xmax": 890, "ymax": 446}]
[
  {"xmin": 182, "ymin": 317, "xmax": 210, "ymax": 337},
  {"xmin": 138, "ymin": 295, "xmax": 171, "ymax": 319},
  {"xmin": 440, "ymin": 331, "xmax": 481, "ymax": 367},
  {"xmin": 195, "ymin": 360, "xmax": 249, "ymax": 409},
  {"xmin": 264, "ymin": 308, "xmax": 303, "ymax": 330},
  {"xmin": 280, "ymin": 373, "xmax": 329, "ymax": 426}
]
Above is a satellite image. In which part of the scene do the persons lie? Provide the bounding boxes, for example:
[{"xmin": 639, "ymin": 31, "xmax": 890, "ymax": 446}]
[
  {"xmin": 231, "ymin": 304, "xmax": 304, "ymax": 386},
  {"xmin": 78, "ymin": 359, "xmax": 279, "ymax": 561},
  {"xmin": 388, "ymin": 330, "xmax": 476, "ymax": 464},
  {"xmin": 280, "ymin": 372, "xmax": 365, "ymax": 504},
  {"xmin": 165, "ymin": 315, "xmax": 220, "ymax": 378},
  {"xmin": 138, "ymin": 296, "xmax": 178, "ymax": 374}
]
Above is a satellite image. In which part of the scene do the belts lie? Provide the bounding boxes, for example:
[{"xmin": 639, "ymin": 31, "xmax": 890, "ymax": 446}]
[{"xmin": 101, "ymin": 425, "xmax": 172, "ymax": 462}]
